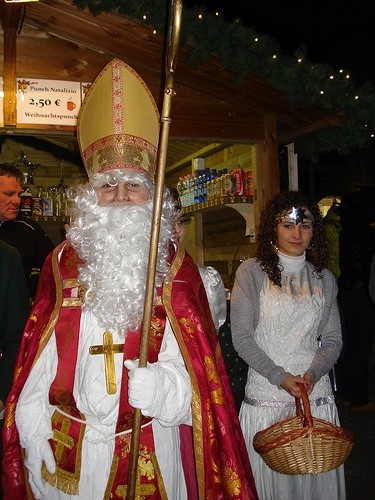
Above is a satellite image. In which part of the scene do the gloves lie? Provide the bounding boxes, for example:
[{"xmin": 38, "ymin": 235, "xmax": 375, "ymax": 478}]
[
  {"xmin": 124, "ymin": 360, "xmax": 158, "ymax": 411},
  {"xmin": 22, "ymin": 438, "xmax": 56, "ymax": 500}
]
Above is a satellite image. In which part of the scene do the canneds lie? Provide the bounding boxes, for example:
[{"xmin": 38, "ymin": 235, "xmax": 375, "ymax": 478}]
[{"xmin": 223, "ymin": 168, "xmax": 254, "ymax": 196}]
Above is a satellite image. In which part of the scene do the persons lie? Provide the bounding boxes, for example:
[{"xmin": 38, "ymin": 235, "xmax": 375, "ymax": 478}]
[
  {"xmin": 170, "ymin": 190, "xmax": 227, "ymax": 335},
  {"xmin": 230, "ymin": 190, "xmax": 346, "ymax": 500},
  {"xmin": 14, "ymin": 142, "xmax": 213, "ymax": 500},
  {"xmin": 0, "ymin": 162, "xmax": 55, "ymax": 421}
]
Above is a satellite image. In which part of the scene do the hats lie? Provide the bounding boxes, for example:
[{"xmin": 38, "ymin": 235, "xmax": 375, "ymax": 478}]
[{"xmin": 76, "ymin": 58, "xmax": 160, "ymax": 179}]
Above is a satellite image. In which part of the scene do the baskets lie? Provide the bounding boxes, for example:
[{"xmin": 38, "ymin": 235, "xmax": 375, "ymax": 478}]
[{"xmin": 253, "ymin": 382, "xmax": 353, "ymax": 475}]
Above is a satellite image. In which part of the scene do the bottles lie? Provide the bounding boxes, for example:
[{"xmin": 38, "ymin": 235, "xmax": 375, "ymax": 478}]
[
  {"xmin": 176, "ymin": 166, "xmax": 230, "ymax": 208},
  {"xmin": 20, "ymin": 182, "xmax": 76, "ymax": 217}
]
